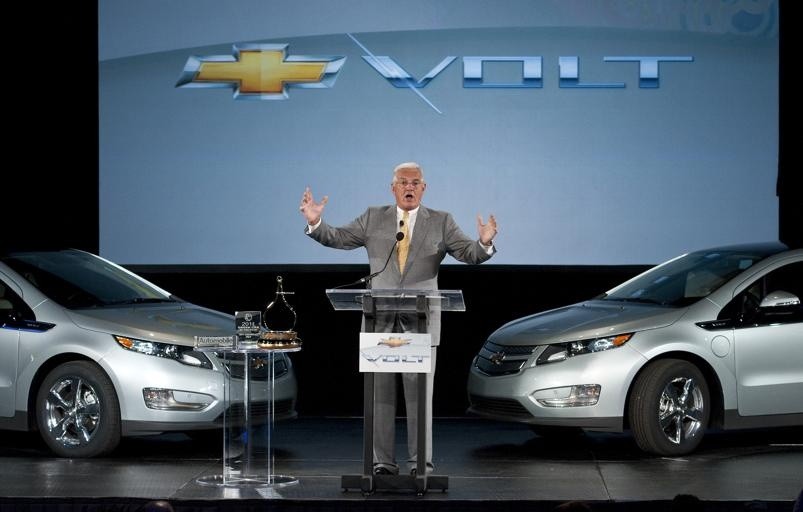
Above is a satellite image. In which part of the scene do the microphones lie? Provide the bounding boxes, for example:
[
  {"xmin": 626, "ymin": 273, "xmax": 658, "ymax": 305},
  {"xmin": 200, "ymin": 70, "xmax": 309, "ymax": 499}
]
[
  {"xmin": 354, "ymin": 232, "xmax": 404, "ymax": 282},
  {"xmin": 399, "ymin": 220, "xmax": 404, "ymax": 226}
]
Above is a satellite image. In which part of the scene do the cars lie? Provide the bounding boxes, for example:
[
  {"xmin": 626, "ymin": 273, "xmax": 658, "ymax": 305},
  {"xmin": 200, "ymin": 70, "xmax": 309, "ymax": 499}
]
[
  {"xmin": 0, "ymin": 247, "xmax": 298, "ymax": 459},
  {"xmin": 464, "ymin": 241, "xmax": 803, "ymax": 458}
]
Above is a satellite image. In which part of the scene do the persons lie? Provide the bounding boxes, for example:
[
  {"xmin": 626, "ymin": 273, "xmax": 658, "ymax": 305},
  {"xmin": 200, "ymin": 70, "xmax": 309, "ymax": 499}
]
[{"xmin": 297, "ymin": 161, "xmax": 498, "ymax": 477}]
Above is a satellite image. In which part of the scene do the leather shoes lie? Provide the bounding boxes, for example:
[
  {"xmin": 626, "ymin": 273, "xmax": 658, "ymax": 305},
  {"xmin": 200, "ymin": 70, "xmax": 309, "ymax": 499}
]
[{"xmin": 373, "ymin": 463, "xmax": 434, "ymax": 477}]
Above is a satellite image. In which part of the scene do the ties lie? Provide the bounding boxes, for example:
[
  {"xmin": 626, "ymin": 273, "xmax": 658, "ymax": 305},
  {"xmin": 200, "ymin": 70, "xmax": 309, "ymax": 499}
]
[{"xmin": 395, "ymin": 211, "xmax": 410, "ymax": 275}]
